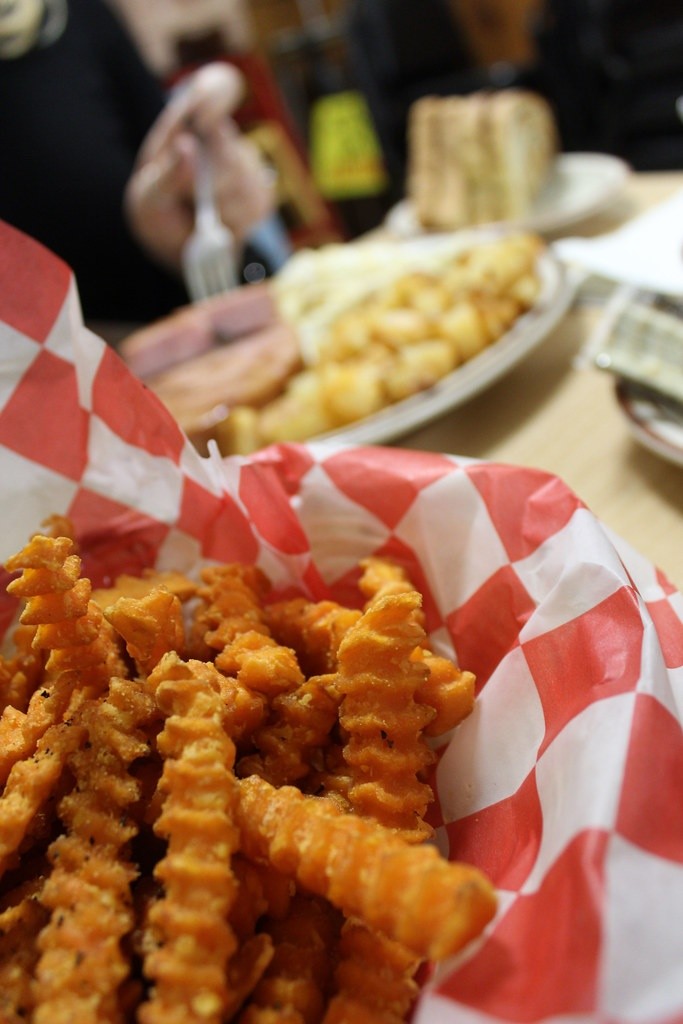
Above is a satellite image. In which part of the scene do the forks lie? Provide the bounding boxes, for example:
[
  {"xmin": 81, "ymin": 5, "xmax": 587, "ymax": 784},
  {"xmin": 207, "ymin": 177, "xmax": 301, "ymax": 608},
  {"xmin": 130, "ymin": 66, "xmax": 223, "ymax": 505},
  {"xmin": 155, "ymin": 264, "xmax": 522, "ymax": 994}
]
[{"xmin": 174, "ymin": 84, "xmax": 236, "ymax": 300}]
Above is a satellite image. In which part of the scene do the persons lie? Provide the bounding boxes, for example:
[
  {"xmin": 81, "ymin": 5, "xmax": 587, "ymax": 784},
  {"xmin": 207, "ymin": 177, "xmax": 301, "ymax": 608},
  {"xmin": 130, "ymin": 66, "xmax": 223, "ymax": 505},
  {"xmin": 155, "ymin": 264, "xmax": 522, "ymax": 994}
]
[
  {"xmin": 0, "ymin": 0, "xmax": 278, "ymax": 326},
  {"xmin": 348, "ymin": 0, "xmax": 683, "ymax": 199}
]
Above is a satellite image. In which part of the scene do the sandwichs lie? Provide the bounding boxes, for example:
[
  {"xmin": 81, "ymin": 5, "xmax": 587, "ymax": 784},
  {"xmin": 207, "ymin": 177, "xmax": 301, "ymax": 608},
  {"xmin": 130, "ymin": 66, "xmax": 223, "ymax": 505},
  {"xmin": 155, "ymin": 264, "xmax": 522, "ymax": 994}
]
[{"xmin": 410, "ymin": 91, "xmax": 548, "ymax": 231}]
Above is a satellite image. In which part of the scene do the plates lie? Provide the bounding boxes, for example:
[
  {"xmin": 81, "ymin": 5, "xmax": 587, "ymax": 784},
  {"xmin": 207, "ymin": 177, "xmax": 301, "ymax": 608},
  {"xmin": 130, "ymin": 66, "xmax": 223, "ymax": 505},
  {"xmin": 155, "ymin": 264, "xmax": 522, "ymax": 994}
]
[
  {"xmin": 383, "ymin": 154, "xmax": 632, "ymax": 233},
  {"xmin": 316, "ymin": 236, "xmax": 574, "ymax": 446},
  {"xmin": 615, "ymin": 378, "xmax": 683, "ymax": 467}
]
